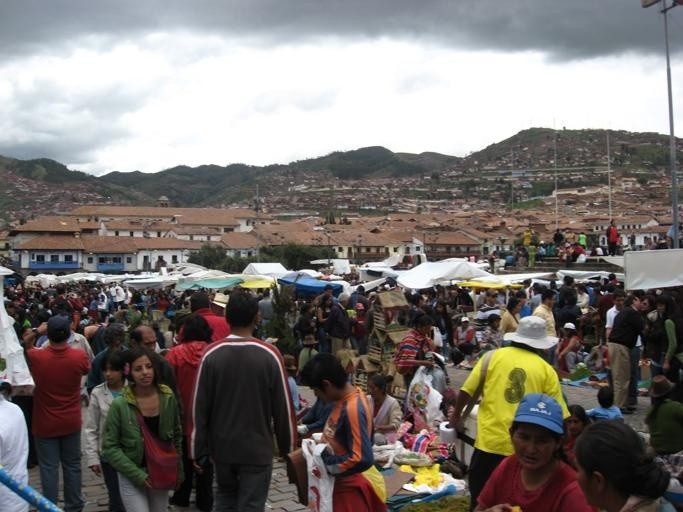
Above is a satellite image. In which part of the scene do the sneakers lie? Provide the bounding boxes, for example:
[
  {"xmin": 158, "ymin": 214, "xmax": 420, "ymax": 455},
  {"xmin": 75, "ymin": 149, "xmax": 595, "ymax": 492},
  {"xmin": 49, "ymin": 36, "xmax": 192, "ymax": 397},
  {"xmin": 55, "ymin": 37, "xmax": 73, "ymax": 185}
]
[{"xmin": 620, "ymin": 408, "xmax": 632, "ymax": 414}]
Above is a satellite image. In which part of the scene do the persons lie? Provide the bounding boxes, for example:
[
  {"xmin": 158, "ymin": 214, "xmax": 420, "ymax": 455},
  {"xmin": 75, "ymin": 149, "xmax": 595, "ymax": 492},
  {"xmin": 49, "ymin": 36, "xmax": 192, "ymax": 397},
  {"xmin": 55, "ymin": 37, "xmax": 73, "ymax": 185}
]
[
  {"xmin": 1, "ymin": 273, "xmax": 682, "ymax": 512},
  {"xmin": 490, "ymin": 219, "xmax": 673, "ymax": 274}
]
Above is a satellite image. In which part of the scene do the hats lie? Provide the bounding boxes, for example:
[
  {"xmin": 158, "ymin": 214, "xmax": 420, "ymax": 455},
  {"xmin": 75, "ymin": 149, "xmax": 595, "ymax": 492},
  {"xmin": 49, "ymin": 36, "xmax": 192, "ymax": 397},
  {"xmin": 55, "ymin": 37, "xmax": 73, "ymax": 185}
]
[
  {"xmin": 283, "ymin": 354, "xmax": 297, "ymax": 370},
  {"xmin": 302, "ymin": 334, "xmax": 318, "ymax": 345},
  {"xmin": 513, "ymin": 393, "xmax": 565, "ymax": 434},
  {"xmin": 209, "ymin": 292, "xmax": 229, "ymax": 309},
  {"xmin": 461, "ymin": 317, "xmax": 469, "ymax": 323},
  {"xmin": 354, "ymin": 302, "xmax": 364, "ymax": 310},
  {"xmin": 563, "ymin": 322, "xmax": 575, "ymax": 329},
  {"xmin": 503, "ymin": 316, "xmax": 560, "ymax": 349},
  {"xmin": 648, "ymin": 374, "xmax": 675, "ymax": 397},
  {"xmin": 47, "ymin": 316, "xmax": 70, "ymax": 338}
]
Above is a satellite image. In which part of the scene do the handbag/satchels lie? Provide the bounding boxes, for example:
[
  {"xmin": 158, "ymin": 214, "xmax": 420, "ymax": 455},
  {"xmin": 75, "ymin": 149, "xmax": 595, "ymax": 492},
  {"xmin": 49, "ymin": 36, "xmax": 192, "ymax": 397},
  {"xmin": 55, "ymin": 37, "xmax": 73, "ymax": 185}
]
[{"xmin": 145, "ymin": 441, "xmax": 178, "ymax": 490}]
